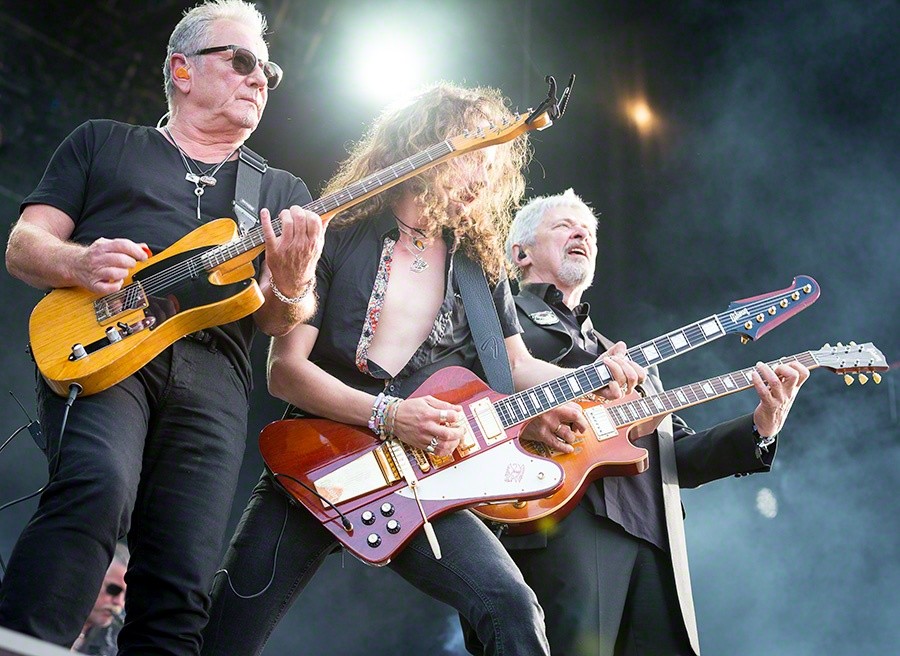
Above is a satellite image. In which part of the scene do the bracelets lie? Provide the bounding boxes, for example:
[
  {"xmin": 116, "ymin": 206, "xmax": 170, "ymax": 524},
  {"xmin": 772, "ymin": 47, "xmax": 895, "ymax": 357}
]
[
  {"xmin": 368, "ymin": 393, "xmax": 404, "ymax": 441},
  {"xmin": 267, "ymin": 274, "xmax": 315, "ymax": 304}
]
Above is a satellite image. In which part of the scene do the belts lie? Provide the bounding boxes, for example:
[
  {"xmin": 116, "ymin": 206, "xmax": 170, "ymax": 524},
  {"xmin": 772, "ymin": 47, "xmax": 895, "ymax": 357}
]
[{"xmin": 185, "ymin": 330, "xmax": 224, "ymax": 352}]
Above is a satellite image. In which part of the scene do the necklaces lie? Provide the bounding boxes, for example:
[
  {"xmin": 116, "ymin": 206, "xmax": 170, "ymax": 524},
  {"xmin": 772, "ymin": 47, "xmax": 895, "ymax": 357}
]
[
  {"xmin": 392, "ymin": 214, "xmax": 444, "ymax": 275},
  {"xmin": 160, "ymin": 124, "xmax": 239, "ymax": 220}
]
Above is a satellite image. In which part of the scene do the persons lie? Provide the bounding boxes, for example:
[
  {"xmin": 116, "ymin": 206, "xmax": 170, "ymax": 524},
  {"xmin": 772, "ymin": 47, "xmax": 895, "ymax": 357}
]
[
  {"xmin": 4, "ymin": 0, "xmax": 326, "ymax": 655},
  {"xmin": 495, "ymin": 188, "xmax": 812, "ymax": 656},
  {"xmin": 208, "ymin": 82, "xmax": 648, "ymax": 656},
  {"xmin": 72, "ymin": 542, "xmax": 133, "ymax": 654}
]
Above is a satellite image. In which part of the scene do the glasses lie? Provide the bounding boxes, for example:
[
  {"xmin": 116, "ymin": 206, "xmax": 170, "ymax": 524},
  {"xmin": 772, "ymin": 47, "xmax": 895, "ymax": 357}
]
[
  {"xmin": 186, "ymin": 45, "xmax": 282, "ymax": 90},
  {"xmin": 102, "ymin": 582, "xmax": 124, "ymax": 597}
]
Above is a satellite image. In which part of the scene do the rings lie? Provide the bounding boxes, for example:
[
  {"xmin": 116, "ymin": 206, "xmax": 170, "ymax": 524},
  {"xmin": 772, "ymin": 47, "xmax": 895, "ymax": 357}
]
[
  {"xmin": 426, "ymin": 446, "xmax": 435, "ymax": 453},
  {"xmin": 440, "ymin": 410, "xmax": 448, "ymax": 424},
  {"xmin": 430, "ymin": 436, "xmax": 439, "ymax": 448},
  {"xmin": 553, "ymin": 424, "xmax": 562, "ymax": 434},
  {"xmin": 621, "ymin": 383, "xmax": 628, "ymax": 392}
]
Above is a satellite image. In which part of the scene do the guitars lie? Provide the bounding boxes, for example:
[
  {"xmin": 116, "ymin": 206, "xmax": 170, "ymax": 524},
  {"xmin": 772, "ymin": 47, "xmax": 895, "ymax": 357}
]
[
  {"xmin": 27, "ymin": 70, "xmax": 583, "ymax": 400},
  {"xmin": 255, "ymin": 273, "xmax": 822, "ymax": 569},
  {"xmin": 466, "ymin": 338, "xmax": 891, "ymax": 538}
]
